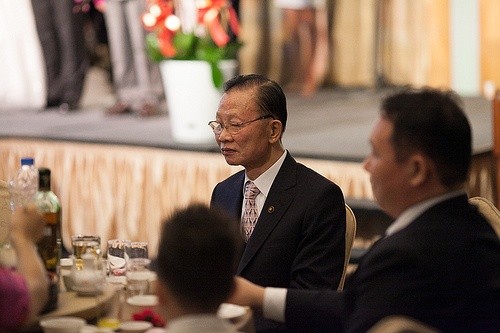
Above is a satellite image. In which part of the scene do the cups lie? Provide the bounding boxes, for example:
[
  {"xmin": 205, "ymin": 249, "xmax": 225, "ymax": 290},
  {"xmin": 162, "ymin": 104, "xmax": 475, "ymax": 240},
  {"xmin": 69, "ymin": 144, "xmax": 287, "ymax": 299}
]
[
  {"xmin": 69, "ymin": 234, "xmax": 102, "ymax": 272},
  {"xmin": 107, "ymin": 238, "xmax": 129, "ymax": 283},
  {"xmin": 126, "ymin": 242, "xmax": 151, "ymax": 291},
  {"xmin": 94, "ymin": 284, "xmax": 123, "ymax": 329},
  {"xmin": 71, "ymin": 269, "xmax": 104, "ymax": 296}
]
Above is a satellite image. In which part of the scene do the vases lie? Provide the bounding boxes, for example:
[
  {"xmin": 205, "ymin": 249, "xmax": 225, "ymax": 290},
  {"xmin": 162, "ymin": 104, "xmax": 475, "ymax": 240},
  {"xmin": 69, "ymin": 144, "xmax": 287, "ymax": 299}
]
[{"xmin": 159, "ymin": 58, "xmax": 238, "ymax": 145}]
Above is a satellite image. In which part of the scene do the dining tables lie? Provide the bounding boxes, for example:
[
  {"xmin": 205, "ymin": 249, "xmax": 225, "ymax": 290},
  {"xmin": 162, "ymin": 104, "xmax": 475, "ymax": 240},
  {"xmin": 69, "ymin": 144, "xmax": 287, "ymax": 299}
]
[{"xmin": 31, "ymin": 254, "xmax": 254, "ymax": 333}]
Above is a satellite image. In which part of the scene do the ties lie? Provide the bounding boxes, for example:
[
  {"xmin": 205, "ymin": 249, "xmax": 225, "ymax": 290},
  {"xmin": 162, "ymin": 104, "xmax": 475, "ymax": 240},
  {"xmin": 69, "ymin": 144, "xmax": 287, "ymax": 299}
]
[{"xmin": 238, "ymin": 181, "xmax": 264, "ymax": 247}]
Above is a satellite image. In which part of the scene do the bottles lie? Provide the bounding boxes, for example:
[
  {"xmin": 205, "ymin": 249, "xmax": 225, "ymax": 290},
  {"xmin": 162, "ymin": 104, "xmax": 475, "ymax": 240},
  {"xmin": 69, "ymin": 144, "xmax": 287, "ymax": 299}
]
[
  {"xmin": 36, "ymin": 224, "xmax": 61, "ymax": 313},
  {"xmin": 15, "ymin": 157, "xmax": 36, "ymax": 207},
  {"xmin": 34, "ymin": 164, "xmax": 62, "ymax": 239}
]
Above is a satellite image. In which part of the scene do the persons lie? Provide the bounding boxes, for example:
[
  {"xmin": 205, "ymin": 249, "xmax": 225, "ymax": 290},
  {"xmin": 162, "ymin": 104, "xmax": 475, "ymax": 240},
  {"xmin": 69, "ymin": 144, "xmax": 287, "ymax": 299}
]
[
  {"xmin": 155, "ymin": 74, "xmax": 347, "ymax": 333},
  {"xmin": 0, "ymin": 204, "xmax": 50, "ymax": 333},
  {"xmin": 226, "ymin": 87, "xmax": 499, "ymax": 333}
]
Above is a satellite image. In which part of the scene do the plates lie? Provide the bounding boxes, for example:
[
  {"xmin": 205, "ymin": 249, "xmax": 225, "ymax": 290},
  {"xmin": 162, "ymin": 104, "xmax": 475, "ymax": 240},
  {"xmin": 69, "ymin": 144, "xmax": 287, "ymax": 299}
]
[
  {"xmin": 219, "ymin": 303, "xmax": 247, "ymax": 319},
  {"xmin": 118, "ymin": 321, "xmax": 151, "ymax": 331},
  {"xmin": 128, "ymin": 295, "xmax": 160, "ymax": 306}
]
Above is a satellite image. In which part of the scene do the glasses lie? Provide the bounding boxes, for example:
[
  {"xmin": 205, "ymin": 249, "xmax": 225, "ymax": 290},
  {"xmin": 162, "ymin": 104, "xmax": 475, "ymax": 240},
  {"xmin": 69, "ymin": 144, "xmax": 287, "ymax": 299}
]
[{"xmin": 208, "ymin": 116, "xmax": 275, "ymax": 134}]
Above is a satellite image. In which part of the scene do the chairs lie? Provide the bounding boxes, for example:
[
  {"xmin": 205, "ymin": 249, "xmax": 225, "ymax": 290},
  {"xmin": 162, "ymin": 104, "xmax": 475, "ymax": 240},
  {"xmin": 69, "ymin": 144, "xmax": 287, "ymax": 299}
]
[
  {"xmin": 469, "ymin": 196, "xmax": 500, "ymax": 239},
  {"xmin": 338, "ymin": 203, "xmax": 356, "ymax": 292}
]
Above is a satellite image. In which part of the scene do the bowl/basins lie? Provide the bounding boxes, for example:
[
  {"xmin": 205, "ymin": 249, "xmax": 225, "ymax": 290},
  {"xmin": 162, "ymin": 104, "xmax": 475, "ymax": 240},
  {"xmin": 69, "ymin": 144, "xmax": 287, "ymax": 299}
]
[
  {"xmin": 40, "ymin": 318, "xmax": 88, "ymax": 333},
  {"xmin": 126, "ymin": 271, "xmax": 156, "ymax": 289}
]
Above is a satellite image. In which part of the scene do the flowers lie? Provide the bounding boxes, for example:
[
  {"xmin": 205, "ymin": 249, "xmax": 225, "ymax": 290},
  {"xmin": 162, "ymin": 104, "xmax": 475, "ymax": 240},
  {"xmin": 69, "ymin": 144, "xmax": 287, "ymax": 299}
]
[{"xmin": 140, "ymin": 0, "xmax": 244, "ymax": 85}]
